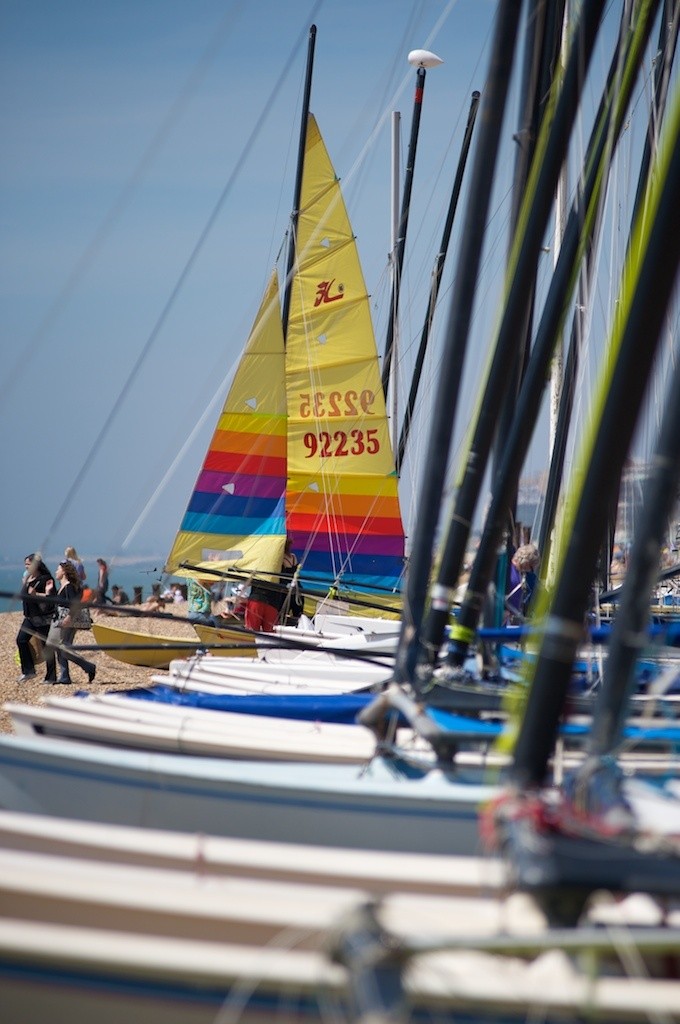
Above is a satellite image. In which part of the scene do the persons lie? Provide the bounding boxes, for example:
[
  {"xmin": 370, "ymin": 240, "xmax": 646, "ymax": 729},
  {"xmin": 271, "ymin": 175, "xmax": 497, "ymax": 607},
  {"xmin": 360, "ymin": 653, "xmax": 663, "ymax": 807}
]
[
  {"xmin": 16, "ymin": 539, "xmax": 305, "ymax": 685},
  {"xmin": 505, "ymin": 543, "xmax": 541, "ymax": 625}
]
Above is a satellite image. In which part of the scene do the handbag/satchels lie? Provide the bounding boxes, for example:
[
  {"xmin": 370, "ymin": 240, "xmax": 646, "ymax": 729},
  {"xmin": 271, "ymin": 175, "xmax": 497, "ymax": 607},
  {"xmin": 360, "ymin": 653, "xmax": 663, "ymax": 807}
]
[
  {"xmin": 29, "ymin": 632, "xmax": 46, "ymax": 664},
  {"xmin": 53, "ymin": 586, "xmax": 92, "ymax": 631}
]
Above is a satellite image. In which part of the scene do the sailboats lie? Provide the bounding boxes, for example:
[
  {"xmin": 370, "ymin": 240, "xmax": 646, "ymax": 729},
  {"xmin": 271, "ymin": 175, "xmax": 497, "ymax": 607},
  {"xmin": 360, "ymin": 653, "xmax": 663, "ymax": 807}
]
[{"xmin": 0, "ymin": 0, "xmax": 680, "ymax": 1024}]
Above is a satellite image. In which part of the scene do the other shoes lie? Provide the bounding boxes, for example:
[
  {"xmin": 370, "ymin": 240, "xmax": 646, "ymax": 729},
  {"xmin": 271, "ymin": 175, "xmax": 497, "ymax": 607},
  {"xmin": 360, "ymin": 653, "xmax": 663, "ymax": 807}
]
[
  {"xmin": 39, "ymin": 679, "xmax": 55, "ymax": 685},
  {"xmin": 18, "ymin": 673, "xmax": 37, "ymax": 682},
  {"xmin": 88, "ymin": 664, "xmax": 96, "ymax": 682},
  {"xmin": 52, "ymin": 680, "xmax": 72, "ymax": 685}
]
